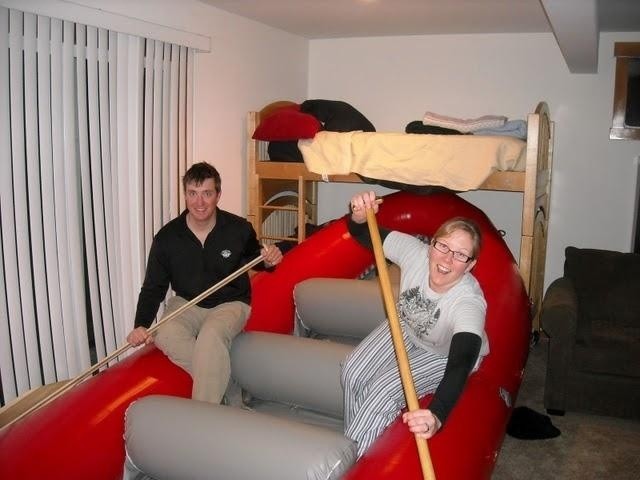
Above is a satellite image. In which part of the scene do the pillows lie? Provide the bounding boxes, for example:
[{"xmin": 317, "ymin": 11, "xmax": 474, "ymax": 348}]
[{"xmin": 252, "ymin": 102, "xmax": 376, "ymax": 142}]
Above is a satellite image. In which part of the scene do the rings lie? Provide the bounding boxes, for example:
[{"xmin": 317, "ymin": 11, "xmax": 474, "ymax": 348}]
[{"xmin": 424, "ymin": 422, "xmax": 430, "ymax": 433}]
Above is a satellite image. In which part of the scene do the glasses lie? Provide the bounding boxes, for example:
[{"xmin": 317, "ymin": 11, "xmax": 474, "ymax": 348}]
[{"xmin": 434, "ymin": 238, "xmax": 472, "ymax": 264}]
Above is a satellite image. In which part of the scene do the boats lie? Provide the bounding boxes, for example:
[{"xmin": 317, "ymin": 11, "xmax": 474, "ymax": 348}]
[{"xmin": 0, "ymin": 190, "xmax": 531, "ymax": 480}]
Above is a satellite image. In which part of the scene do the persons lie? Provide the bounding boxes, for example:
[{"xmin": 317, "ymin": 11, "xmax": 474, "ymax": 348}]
[
  {"xmin": 125, "ymin": 162, "xmax": 286, "ymax": 405},
  {"xmin": 340, "ymin": 188, "xmax": 492, "ymax": 464}
]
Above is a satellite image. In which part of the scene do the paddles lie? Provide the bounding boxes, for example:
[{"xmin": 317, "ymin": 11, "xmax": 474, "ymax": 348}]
[{"xmin": 0, "ymin": 243, "xmax": 277, "ymax": 436}]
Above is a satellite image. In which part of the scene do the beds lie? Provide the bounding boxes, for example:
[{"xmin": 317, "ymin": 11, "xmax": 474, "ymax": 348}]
[{"xmin": 246, "ymin": 100, "xmax": 557, "ymax": 335}]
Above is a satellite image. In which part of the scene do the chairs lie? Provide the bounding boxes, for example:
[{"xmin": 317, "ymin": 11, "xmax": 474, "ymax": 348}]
[{"xmin": 539, "ymin": 246, "xmax": 638, "ymax": 416}]
